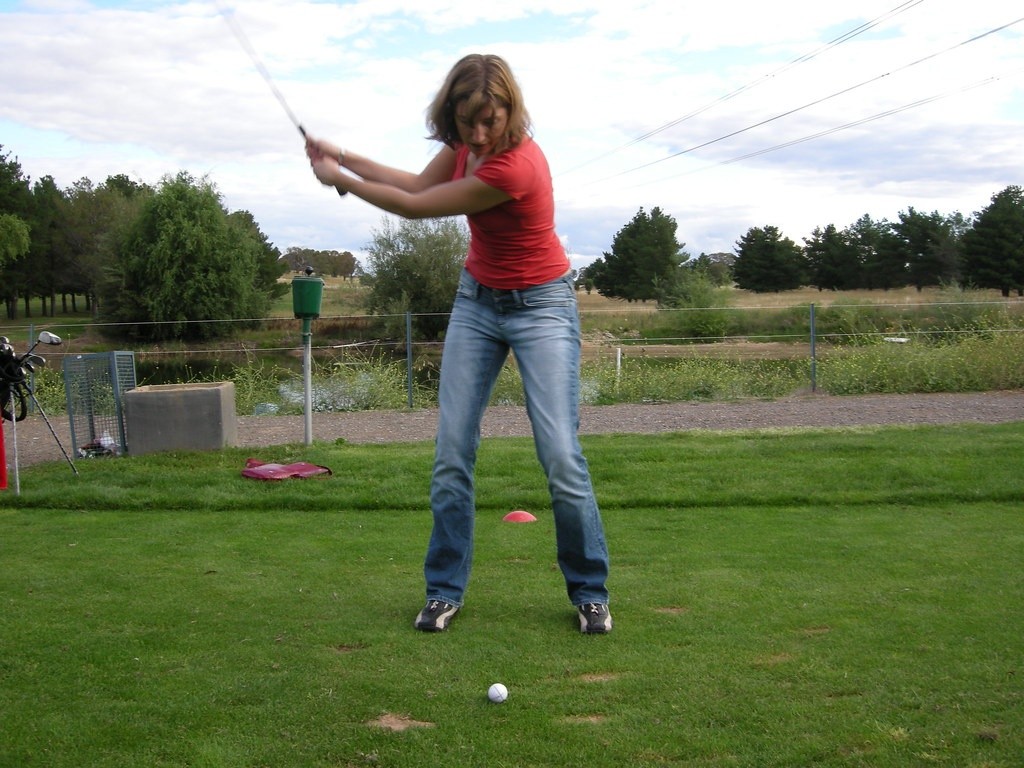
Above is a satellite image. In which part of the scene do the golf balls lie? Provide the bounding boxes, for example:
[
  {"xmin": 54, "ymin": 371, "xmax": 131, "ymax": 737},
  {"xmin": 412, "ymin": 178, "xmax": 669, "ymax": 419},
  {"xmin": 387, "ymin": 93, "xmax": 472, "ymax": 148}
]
[{"xmin": 487, "ymin": 682, "xmax": 509, "ymax": 703}]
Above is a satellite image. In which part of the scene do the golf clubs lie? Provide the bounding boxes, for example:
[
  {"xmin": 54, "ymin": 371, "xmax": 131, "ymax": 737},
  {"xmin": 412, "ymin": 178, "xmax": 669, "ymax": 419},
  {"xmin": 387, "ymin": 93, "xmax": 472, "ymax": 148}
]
[
  {"xmin": 1, "ymin": 330, "xmax": 82, "ymax": 496},
  {"xmin": 214, "ymin": 1, "xmax": 350, "ymax": 198}
]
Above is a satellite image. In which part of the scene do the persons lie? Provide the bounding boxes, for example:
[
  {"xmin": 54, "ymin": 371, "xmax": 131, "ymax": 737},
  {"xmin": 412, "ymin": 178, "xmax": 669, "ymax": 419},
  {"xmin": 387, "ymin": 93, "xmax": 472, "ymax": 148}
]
[{"xmin": 305, "ymin": 54, "xmax": 613, "ymax": 635}]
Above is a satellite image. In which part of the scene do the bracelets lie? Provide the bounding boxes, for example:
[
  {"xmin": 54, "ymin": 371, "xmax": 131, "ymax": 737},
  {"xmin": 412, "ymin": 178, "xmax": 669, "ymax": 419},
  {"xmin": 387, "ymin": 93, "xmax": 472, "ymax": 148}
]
[{"xmin": 337, "ymin": 149, "xmax": 345, "ymax": 165}]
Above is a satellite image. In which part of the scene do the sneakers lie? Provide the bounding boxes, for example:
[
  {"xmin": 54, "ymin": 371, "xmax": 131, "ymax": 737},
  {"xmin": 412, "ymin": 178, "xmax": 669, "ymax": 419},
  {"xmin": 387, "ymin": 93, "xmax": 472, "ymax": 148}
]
[
  {"xmin": 578, "ymin": 604, "xmax": 613, "ymax": 633},
  {"xmin": 414, "ymin": 598, "xmax": 459, "ymax": 631}
]
